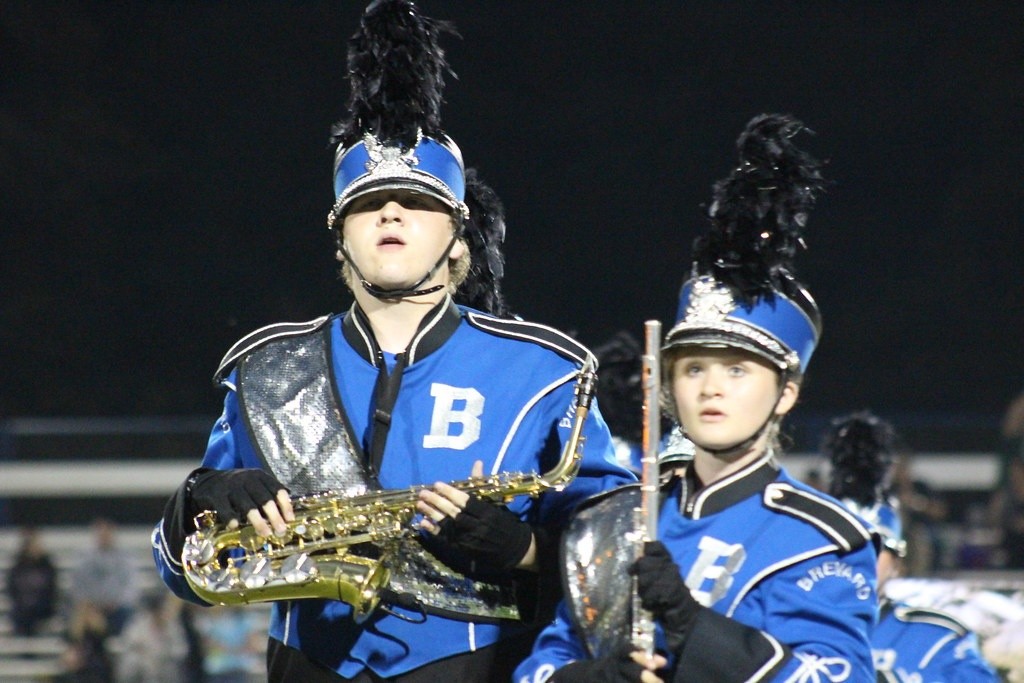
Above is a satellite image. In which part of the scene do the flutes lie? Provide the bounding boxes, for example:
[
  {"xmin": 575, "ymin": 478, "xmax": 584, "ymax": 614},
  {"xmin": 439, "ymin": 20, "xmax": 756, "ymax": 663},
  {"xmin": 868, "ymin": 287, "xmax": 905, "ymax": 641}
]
[{"xmin": 620, "ymin": 316, "xmax": 668, "ymax": 662}]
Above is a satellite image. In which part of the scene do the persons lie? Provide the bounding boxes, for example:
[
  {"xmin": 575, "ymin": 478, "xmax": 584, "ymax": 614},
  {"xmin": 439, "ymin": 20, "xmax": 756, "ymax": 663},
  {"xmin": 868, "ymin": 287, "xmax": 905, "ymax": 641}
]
[
  {"xmin": 147, "ymin": 0, "xmax": 639, "ymax": 683},
  {"xmin": 825, "ymin": 386, "xmax": 1024, "ymax": 682},
  {"xmin": 507, "ymin": 113, "xmax": 877, "ymax": 683},
  {"xmin": 8, "ymin": 517, "xmax": 266, "ymax": 683}
]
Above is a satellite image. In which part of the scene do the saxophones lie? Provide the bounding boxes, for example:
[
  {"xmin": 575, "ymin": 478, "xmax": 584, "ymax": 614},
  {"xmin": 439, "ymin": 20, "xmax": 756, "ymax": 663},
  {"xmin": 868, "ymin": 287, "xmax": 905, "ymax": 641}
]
[{"xmin": 179, "ymin": 358, "xmax": 600, "ymax": 627}]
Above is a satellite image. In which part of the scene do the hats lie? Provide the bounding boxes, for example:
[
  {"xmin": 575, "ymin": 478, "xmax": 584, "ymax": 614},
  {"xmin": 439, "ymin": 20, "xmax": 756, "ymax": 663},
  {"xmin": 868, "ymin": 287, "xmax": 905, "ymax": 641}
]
[
  {"xmin": 326, "ymin": 125, "xmax": 470, "ymax": 233},
  {"xmin": 831, "ymin": 490, "xmax": 906, "ymax": 557},
  {"xmin": 660, "ymin": 264, "xmax": 822, "ymax": 383}
]
